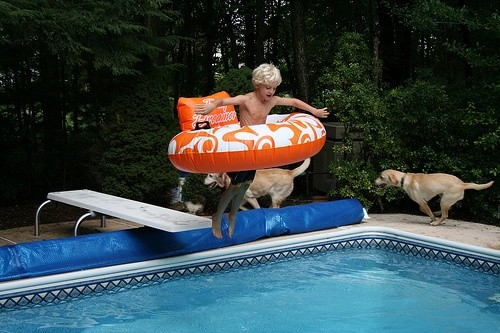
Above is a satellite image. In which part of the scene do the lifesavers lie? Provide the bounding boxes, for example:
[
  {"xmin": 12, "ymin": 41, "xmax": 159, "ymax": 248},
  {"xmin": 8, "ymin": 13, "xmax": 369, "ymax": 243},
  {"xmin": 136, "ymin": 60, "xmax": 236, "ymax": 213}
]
[{"xmin": 167, "ymin": 91, "xmax": 325, "ymax": 173}]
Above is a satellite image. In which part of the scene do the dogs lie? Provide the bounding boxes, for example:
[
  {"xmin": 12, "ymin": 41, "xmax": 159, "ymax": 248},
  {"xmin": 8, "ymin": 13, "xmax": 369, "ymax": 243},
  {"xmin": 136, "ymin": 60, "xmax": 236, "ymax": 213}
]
[
  {"xmin": 374, "ymin": 169, "xmax": 495, "ymax": 227},
  {"xmin": 201, "ymin": 158, "xmax": 312, "ymax": 212}
]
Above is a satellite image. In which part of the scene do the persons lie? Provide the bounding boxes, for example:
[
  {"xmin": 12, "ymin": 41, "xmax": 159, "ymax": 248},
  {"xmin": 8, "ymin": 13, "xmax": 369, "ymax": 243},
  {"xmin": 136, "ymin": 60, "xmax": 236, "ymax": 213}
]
[{"xmin": 194, "ymin": 63, "xmax": 329, "ymax": 238}]
